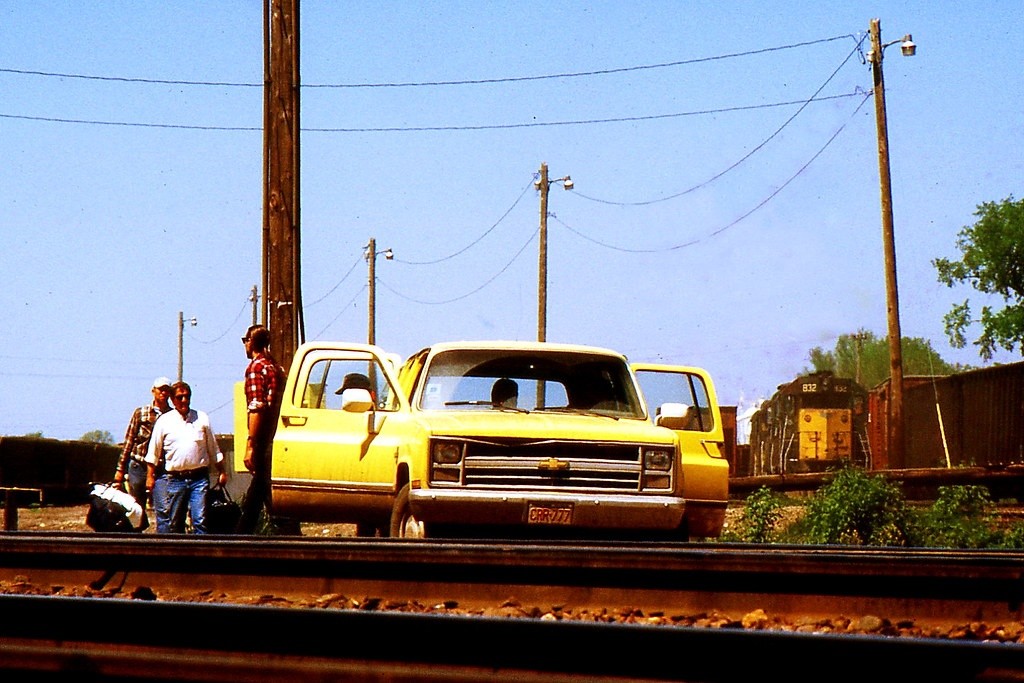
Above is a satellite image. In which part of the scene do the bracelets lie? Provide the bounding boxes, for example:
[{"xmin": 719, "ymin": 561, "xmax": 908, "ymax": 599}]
[{"xmin": 247, "ymin": 436, "xmax": 256, "ymax": 440}]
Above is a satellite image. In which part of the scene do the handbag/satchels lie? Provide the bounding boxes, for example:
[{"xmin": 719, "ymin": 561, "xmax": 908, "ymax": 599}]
[
  {"xmin": 204, "ymin": 484, "xmax": 242, "ymax": 534},
  {"xmin": 86, "ymin": 480, "xmax": 149, "ymax": 532}
]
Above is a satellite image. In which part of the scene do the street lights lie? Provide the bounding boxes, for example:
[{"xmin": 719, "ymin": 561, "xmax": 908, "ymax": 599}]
[
  {"xmin": 362, "ymin": 239, "xmax": 394, "ymax": 397},
  {"xmin": 533, "ymin": 160, "xmax": 574, "ymax": 408},
  {"xmin": 177, "ymin": 310, "xmax": 198, "ymax": 384},
  {"xmin": 869, "ymin": 17, "xmax": 919, "ymax": 467}
]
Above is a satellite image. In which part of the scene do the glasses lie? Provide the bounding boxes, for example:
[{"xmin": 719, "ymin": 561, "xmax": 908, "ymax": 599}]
[
  {"xmin": 242, "ymin": 338, "xmax": 248, "ymax": 344},
  {"xmin": 173, "ymin": 394, "xmax": 191, "ymax": 400}
]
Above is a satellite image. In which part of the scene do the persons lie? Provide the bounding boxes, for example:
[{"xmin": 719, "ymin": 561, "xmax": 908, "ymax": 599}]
[
  {"xmin": 236, "ymin": 326, "xmax": 302, "ymax": 534},
  {"xmin": 144, "ymin": 381, "xmax": 227, "ymax": 533},
  {"xmin": 335, "ymin": 374, "xmax": 380, "ymax": 537},
  {"xmin": 114, "ymin": 376, "xmax": 175, "ymax": 532},
  {"xmin": 491, "ymin": 378, "xmax": 518, "ymax": 408}
]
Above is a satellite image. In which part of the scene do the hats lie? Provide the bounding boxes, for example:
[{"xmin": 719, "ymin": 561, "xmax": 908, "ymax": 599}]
[
  {"xmin": 335, "ymin": 373, "xmax": 370, "ymax": 395},
  {"xmin": 154, "ymin": 377, "xmax": 172, "ymax": 388}
]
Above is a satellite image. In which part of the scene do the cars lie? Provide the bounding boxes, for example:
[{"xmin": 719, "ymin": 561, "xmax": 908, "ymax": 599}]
[{"xmin": 233, "ymin": 341, "xmax": 729, "ymax": 541}]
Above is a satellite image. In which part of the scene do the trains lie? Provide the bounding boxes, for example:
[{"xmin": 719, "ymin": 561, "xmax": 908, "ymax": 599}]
[{"xmin": 749, "ymin": 369, "xmax": 869, "ymax": 475}]
